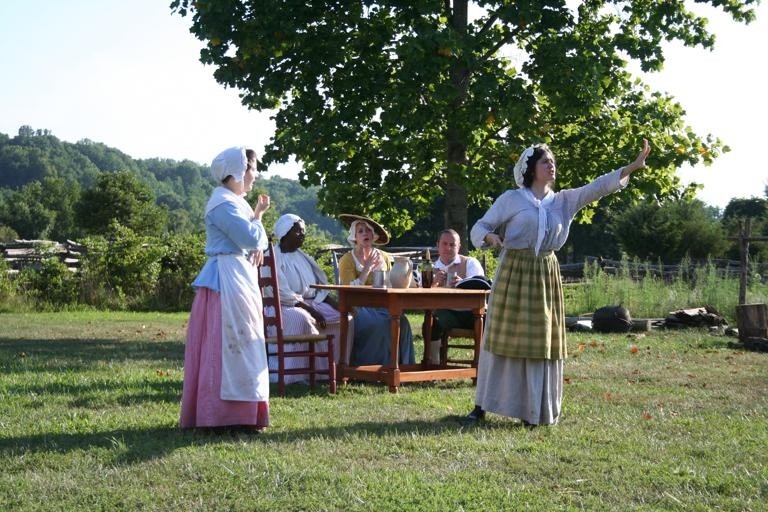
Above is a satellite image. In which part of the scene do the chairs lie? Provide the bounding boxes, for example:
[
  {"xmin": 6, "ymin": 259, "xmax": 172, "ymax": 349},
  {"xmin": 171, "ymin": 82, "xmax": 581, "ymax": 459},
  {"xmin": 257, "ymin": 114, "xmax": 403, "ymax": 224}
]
[
  {"xmin": 257, "ymin": 232, "xmax": 337, "ymax": 399},
  {"xmin": 440, "ymin": 254, "xmax": 485, "ymax": 367}
]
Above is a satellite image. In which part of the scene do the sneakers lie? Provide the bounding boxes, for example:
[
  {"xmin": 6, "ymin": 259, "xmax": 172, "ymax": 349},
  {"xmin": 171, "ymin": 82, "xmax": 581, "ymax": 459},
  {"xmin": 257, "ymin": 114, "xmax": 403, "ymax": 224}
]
[{"xmin": 466, "ymin": 405, "xmax": 486, "ymax": 422}]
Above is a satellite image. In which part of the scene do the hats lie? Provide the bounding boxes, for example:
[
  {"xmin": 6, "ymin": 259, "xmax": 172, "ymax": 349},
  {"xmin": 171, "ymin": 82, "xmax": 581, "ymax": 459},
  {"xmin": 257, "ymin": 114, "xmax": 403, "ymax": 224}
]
[
  {"xmin": 273, "ymin": 214, "xmax": 307, "ymax": 242},
  {"xmin": 337, "ymin": 211, "xmax": 389, "ymax": 245},
  {"xmin": 455, "ymin": 274, "xmax": 493, "ymax": 292},
  {"xmin": 210, "ymin": 145, "xmax": 248, "ymax": 185},
  {"xmin": 513, "ymin": 142, "xmax": 551, "ymax": 189}
]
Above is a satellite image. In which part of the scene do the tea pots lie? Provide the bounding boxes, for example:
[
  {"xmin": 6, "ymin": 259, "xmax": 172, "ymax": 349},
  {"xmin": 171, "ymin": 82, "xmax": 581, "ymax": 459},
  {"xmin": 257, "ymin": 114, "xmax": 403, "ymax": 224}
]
[{"xmin": 389, "ymin": 255, "xmax": 414, "ymax": 289}]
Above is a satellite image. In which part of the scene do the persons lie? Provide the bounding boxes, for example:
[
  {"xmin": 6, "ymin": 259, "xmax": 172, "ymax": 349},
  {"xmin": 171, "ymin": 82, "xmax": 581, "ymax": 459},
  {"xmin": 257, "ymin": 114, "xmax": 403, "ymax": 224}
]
[
  {"xmin": 338, "ymin": 214, "xmax": 415, "ymax": 367},
  {"xmin": 181, "ymin": 146, "xmax": 269, "ymax": 433},
  {"xmin": 422, "ymin": 229, "xmax": 485, "ymax": 364},
  {"xmin": 468, "ymin": 138, "xmax": 650, "ymax": 431},
  {"xmin": 260, "ymin": 214, "xmax": 355, "ymax": 386}
]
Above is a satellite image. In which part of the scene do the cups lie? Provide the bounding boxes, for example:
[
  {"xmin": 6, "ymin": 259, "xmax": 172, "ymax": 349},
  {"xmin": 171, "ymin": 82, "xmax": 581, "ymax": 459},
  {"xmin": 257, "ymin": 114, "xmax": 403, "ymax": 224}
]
[
  {"xmin": 374, "ymin": 269, "xmax": 421, "ymax": 287},
  {"xmin": 446, "ymin": 272, "xmax": 458, "ymax": 288}
]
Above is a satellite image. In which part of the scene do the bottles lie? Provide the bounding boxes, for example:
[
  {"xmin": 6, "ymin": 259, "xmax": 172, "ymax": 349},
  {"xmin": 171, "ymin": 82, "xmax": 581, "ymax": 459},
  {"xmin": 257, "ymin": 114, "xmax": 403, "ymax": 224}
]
[{"xmin": 421, "ymin": 259, "xmax": 433, "ymax": 288}]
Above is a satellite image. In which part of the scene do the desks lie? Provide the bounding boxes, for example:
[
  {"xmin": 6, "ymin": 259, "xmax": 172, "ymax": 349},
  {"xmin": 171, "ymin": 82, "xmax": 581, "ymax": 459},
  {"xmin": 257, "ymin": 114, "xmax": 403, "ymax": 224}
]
[{"xmin": 309, "ymin": 284, "xmax": 491, "ymax": 394}]
